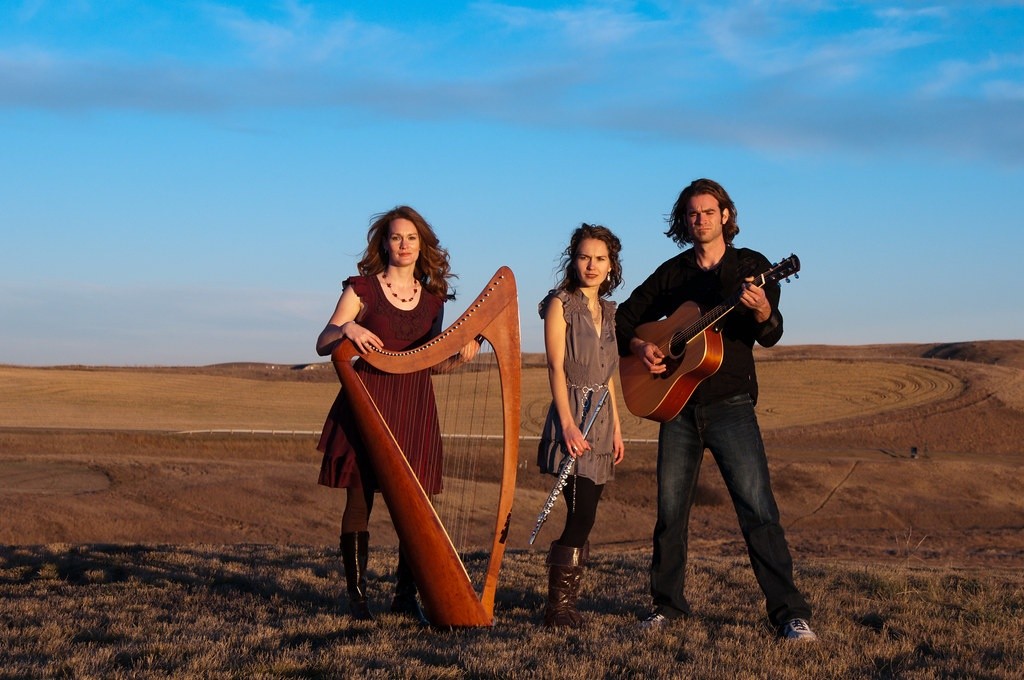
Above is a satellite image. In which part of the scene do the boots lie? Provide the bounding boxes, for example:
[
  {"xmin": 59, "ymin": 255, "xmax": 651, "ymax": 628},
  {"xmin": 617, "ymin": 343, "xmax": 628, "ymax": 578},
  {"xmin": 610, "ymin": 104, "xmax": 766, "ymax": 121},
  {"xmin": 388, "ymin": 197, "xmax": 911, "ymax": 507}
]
[
  {"xmin": 340, "ymin": 530, "xmax": 374, "ymax": 620},
  {"xmin": 391, "ymin": 542, "xmax": 430, "ymax": 626},
  {"xmin": 545, "ymin": 539, "xmax": 589, "ymax": 630}
]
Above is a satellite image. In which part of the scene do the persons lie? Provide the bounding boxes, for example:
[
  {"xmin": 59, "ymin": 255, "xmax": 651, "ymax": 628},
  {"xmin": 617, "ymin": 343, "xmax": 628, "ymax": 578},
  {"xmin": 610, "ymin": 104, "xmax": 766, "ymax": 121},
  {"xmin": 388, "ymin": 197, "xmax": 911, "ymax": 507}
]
[
  {"xmin": 537, "ymin": 223, "xmax": 624, "ymax": 630},
  {"xmin": 614, "ymin": 179, "xmax": 817, "ymax": 640},
  {"xmin": 316, "ymin": 207, "xmax": 480, "ymax": 626}
]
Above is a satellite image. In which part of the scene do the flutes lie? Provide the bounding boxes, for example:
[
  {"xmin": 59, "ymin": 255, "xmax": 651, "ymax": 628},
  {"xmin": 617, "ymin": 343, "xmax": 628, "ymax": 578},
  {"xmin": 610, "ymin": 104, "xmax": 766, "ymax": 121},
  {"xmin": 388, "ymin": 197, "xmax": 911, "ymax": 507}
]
[{"xmin": 528, "ymin": 388, "xmax": 610, "ymax": 546}]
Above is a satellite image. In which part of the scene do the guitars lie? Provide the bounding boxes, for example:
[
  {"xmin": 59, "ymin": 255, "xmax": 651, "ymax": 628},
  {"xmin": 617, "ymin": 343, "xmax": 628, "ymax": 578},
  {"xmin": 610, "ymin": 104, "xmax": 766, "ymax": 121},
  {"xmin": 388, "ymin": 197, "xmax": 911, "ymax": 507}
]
[{"xmin": 618, "ymin": 251, "xmax": 802, "ymax": 424}]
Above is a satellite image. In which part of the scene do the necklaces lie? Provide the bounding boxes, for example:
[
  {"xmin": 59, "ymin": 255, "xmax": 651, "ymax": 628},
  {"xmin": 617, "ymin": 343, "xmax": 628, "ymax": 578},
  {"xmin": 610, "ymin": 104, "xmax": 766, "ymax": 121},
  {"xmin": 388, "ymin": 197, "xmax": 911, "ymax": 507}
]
[
  {"xmin": 383, "ymin": 272, "xmax": 418, "ymax": 302},
  {"xmin": 592, "ymin": 300, "xmax": 601, "ymax": 324}
]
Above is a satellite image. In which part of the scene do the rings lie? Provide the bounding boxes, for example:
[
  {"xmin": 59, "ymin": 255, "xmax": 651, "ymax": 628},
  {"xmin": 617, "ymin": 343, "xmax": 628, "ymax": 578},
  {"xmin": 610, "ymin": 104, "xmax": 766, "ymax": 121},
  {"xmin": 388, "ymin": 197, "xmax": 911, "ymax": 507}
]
[{"xmin": 573, "ymin": 447, "xmax": 578, "ymax": 452}]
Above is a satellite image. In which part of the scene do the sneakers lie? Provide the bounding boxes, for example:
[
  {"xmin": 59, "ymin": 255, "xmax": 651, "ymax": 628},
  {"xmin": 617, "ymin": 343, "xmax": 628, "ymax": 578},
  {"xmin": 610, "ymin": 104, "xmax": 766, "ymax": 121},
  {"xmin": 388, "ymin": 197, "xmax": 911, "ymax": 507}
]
[
  {"xmin": 639, "ymin": 613, "xmax": 665, "ymax": 633},
  {"xmin": 783, "ymin": 617, "xmax": 817, "ymax": 641}
]
